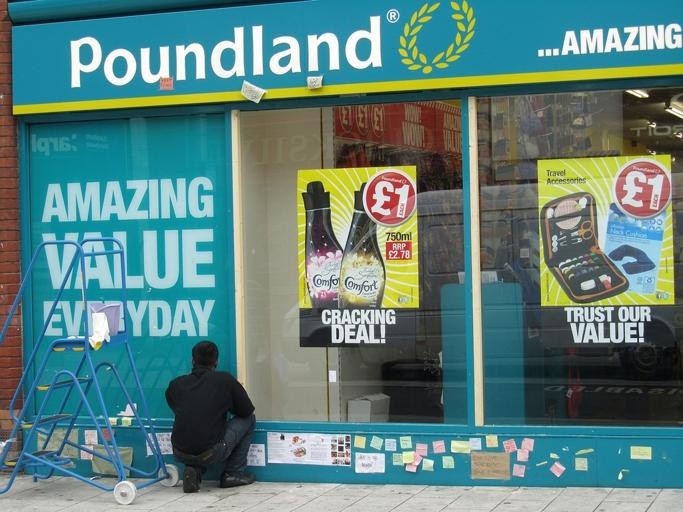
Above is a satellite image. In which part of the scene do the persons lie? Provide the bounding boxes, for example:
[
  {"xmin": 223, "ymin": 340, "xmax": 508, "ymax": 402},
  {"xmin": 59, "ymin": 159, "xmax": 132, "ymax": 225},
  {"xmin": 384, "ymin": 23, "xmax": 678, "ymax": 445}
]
[{"xmin": 165, "ymin": 340, "xmax": 257, "ymax": 492}]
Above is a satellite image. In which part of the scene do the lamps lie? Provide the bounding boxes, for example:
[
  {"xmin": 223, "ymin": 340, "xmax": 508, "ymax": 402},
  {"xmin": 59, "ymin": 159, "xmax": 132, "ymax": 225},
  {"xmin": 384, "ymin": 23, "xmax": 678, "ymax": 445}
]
[
  {"xmin": 664, "ymin": 102, "xmax": 683, "ymax": 119},
  {"xmin": 625, "ymin": 89, "xmax": 650, "ymax": 99}
]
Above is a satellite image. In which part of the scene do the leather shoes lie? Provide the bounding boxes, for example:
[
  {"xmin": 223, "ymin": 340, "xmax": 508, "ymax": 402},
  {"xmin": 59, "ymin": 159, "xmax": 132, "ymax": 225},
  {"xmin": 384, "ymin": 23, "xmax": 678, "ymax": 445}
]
[
  {"xmin": 183, "ymin": 467, "xmax": 199, "ymax": 493},
  {"xmin": 220, "ymin": 471, "xmax": 256, "ymax": 488}
]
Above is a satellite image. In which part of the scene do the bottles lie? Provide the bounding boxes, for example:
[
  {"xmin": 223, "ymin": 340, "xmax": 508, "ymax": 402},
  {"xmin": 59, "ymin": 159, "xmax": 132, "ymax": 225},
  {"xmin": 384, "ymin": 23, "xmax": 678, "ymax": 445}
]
[{"xmin": 301, "ymin": 180, "xmax": 386, "ymax": 309}]
